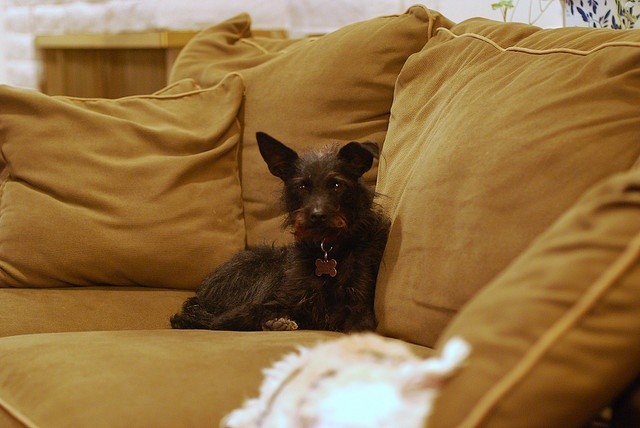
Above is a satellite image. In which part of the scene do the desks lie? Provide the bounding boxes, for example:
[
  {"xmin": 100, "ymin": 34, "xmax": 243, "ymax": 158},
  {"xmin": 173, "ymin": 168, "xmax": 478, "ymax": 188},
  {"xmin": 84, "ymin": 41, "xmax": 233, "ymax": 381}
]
[{"xmin": 33, "ymin": 30, "xmax": 287, "ymax": 100}]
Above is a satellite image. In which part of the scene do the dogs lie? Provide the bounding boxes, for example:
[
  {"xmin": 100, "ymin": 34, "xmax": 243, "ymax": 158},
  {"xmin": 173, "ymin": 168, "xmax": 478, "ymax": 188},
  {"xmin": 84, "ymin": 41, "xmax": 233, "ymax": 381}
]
[{"xmin": 170, "ymin": 129, "xmax": 395, "ymax": 335}]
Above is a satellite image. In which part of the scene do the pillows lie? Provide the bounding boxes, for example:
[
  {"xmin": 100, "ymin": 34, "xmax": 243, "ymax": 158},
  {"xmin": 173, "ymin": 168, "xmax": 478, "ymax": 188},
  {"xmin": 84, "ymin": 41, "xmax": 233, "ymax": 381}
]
[
  {"xmin": 168, "ymin": 2, "xmax": 447, "ymax": 254},
  {"xmin": 0, "ymin": 72, "xmax": 246, "ymax": 290},
  {"xmin": 424, "ymin": 161, "xmax": 639, "ymax": 426},
  {"xmin": 370, "ymin": 16, "xmax": 639, "ymax": 350}
]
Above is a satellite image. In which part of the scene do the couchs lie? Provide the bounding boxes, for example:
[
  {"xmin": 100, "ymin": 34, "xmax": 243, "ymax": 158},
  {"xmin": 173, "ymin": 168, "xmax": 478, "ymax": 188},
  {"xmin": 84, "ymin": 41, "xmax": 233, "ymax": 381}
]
[{"xmin": 0, "ymin": 4, "xmax": 639, "ymax": 427}]
